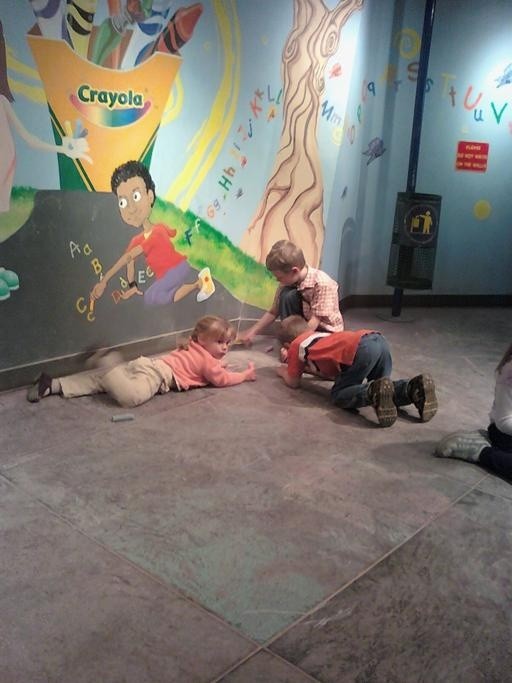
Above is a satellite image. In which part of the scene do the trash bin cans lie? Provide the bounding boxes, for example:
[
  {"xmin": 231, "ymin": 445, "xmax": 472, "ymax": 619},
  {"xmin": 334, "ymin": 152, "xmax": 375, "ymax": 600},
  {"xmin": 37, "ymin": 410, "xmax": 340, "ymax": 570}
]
[{"xmin": 387, "ymin": 190, "xmax": 443, "ymax": 292}]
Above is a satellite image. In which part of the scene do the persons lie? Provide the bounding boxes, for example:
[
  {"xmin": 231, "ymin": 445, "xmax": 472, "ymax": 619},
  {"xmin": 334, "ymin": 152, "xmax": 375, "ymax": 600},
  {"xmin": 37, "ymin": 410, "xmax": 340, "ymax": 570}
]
[
  {"xmin": 1, "ymin": 25, "xmax": 91, "ymax": 299},
  {"xmin": 275, "ymin": 315, "xmax": 439, "ymax": 428},
  {"xmin": 233, "ymin": 241, "xmax": 339, "ymax": 366},
  {"xmin": 434, "ymin": 348, "xmax": 512, "ymax": 485},
  {"xmin": 24, "ymin": 316, "xmax": 257, "ymax": 408},
  {"xmin": 89, "ymin": 160, "xmax": 212, "ymax": 313}
]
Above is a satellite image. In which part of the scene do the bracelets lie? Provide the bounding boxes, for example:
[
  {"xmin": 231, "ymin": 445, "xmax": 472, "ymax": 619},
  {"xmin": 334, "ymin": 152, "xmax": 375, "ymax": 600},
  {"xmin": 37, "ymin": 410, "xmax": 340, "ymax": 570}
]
[{"xmin": 129, "ymin": 282, "xmax": 137, "ymax": 287}]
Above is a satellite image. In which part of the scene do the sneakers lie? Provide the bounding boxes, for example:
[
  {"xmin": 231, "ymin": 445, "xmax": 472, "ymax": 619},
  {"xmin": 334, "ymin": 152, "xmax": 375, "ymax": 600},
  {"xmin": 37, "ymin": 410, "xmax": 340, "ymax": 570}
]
[
  {"xmin": 369, "ymin": 378, "xmax": 396, "ymax": 427},
  {"xmin": 436, "ymin": 430, "xmax": 489, "ymax": 462},
  {"xmin": 408, "ymin": 374, "xmax": 437, "ymax": 422},
  {"xmin": 28, "ymin": 373, "xmax": 52, "ymax": 400}
]
